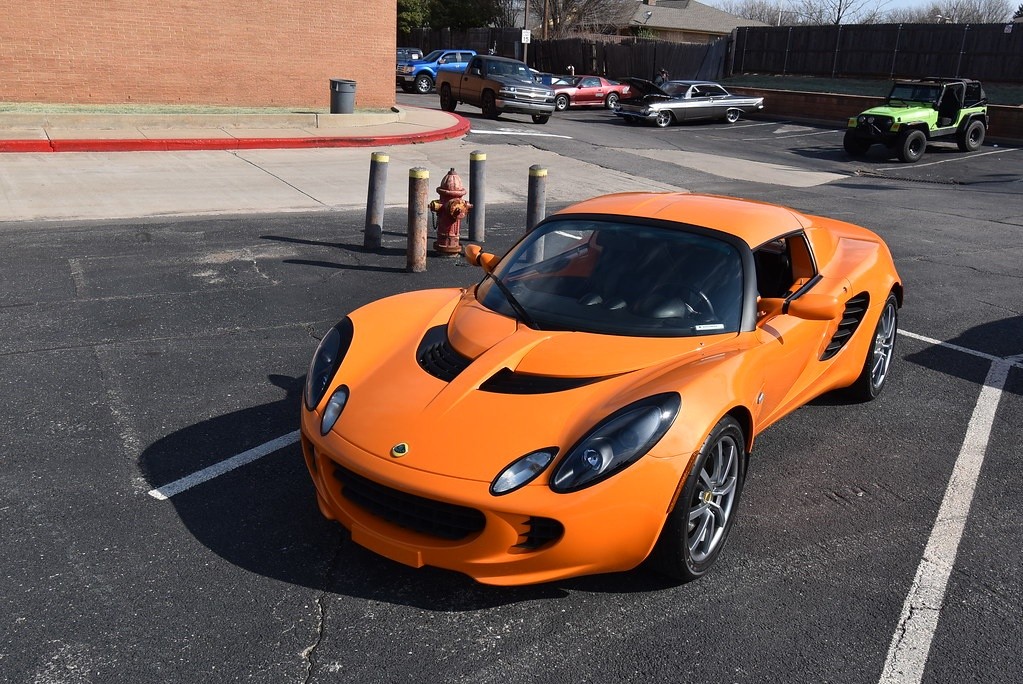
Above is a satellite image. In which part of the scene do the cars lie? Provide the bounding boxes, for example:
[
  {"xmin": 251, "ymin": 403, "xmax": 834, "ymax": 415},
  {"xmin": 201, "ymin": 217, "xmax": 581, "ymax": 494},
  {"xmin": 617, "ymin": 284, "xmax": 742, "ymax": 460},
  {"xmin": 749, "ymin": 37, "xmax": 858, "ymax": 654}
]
[
  {"xmin": 548, "ymin": 74, "xmax": 632, "ymax": 111},
  {"xmin": 517, "ymin": 67, "xmax": 569, "ymax": 92}
]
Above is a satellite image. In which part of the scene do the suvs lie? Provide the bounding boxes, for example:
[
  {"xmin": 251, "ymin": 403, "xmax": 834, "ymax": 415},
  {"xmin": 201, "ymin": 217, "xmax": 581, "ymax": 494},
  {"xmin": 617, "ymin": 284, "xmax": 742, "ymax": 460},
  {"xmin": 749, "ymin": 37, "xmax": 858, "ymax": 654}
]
[{"xmin": 842, "ymin": 76, "xmax": 991, "ymax": 166}]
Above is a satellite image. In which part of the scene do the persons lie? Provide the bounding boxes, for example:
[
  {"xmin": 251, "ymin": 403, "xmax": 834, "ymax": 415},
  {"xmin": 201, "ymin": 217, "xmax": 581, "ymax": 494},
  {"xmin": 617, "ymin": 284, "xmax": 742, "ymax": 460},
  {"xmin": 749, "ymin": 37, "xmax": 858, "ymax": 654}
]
[{"xmin": 651, "ymin": 71, "xmax": 669, "ymax": 88}]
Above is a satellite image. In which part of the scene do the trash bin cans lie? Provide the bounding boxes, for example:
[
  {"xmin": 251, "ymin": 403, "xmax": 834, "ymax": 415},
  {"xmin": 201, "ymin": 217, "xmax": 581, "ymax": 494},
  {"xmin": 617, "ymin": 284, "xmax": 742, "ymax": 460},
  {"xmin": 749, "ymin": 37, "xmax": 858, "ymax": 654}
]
[{"xmin": 329, "ymin": 78, "xmax": 357, "ymax": 114}]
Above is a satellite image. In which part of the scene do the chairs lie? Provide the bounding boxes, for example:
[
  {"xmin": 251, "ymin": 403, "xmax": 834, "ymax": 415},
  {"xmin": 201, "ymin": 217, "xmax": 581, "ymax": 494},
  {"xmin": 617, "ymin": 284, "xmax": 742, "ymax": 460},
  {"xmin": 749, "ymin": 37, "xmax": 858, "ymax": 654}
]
[{"xmin": 564, "ymin": 228, "xmax": 793, "ymax": 323}]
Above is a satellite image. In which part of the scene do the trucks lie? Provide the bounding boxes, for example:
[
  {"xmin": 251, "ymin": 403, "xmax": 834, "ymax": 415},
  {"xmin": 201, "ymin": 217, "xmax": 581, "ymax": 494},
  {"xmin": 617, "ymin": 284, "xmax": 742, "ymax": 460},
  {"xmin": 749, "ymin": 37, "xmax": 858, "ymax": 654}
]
[{"xmin": 396, "ymin": 48, "xmax": 423, "ymax": 87}]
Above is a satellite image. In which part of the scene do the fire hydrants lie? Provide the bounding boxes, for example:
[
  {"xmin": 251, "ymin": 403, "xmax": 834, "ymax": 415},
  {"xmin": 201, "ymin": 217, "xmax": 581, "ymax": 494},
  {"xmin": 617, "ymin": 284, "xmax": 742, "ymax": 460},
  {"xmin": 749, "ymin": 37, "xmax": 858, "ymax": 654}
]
[{"xmin": 426, "ymin": 167, "xmax": 474, "ymax": 257}]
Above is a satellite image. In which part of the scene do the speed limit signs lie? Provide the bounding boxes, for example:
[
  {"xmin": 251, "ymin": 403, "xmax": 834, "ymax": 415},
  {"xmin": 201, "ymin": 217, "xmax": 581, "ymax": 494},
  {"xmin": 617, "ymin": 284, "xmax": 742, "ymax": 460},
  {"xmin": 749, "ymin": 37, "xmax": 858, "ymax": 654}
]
[{"xmin": 522, "ymin": 29, "xmax": 532, "ymax": 44}]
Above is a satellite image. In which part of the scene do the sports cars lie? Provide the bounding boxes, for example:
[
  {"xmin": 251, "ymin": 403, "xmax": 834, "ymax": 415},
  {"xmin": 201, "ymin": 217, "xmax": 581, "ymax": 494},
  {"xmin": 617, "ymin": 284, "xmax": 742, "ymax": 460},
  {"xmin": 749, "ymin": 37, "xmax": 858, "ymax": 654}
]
[{"xmin": 296, "ymin": 191, "xmax": 916, "ymax": 593}]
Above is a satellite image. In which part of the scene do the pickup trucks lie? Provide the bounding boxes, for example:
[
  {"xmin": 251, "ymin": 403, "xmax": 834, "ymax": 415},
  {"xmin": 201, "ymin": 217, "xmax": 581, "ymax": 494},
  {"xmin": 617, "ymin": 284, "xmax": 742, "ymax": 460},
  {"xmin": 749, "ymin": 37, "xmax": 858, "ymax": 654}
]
[
  {"xmin": 613, "ymin": 76, "xmax": 765, "ymax": 128},
  {"xmin": 397, "ymin": 49, "xmax": 513, "ymax": 97},
  {"xmin": 436, "ymin": 54, "xmax": 557, "ymax": 124}
]
